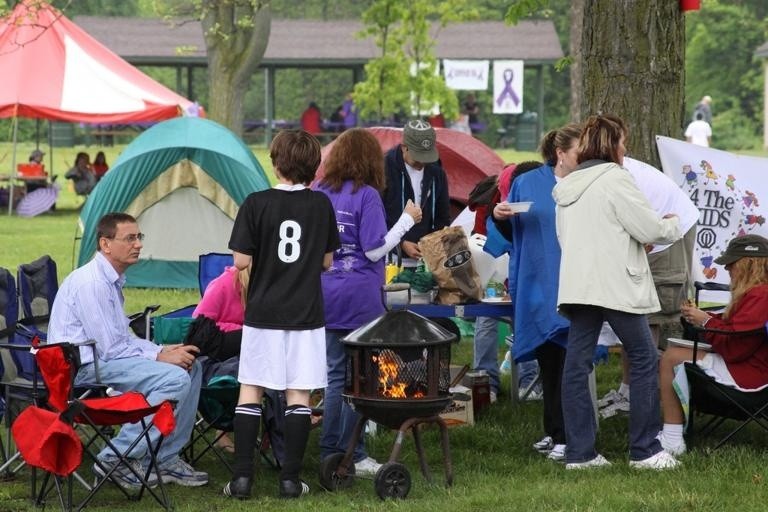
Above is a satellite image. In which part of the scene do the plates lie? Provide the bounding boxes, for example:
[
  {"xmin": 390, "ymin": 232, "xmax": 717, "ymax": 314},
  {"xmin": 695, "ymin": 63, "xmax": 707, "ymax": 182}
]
[{"xmin": 666, "ymin": 337, "xmax": 714, "ymax": 352}]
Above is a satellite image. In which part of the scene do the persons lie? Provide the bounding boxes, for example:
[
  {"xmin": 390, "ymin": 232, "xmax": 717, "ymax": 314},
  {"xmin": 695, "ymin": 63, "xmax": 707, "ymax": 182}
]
[
  {"xmin": 329, "ymin": 106, "xmax": 345, "ymax": 139},
  {"xmin": 382, "ymin": 120, "xmax": 451, "ymax": 270},
  {"xmin": 428, "ymin": 106, "xmax": 445, "ymax": 128},
  {"xmin": 29, "ymin": 150, "xmax": 46, "ymax": 165},
  {"xmin": 552, "ymin": 116, "xmax": 683, "ymax": 470},
  {"xmin": 309, "ymin": 128, "xmax": 422, "ymax": 476},
  {"xmin": 221, "ymin": 129, "xmax": 341, "ymax": 498},
  {"xmin": 598, "ymin": 158, "xmax": 701, "ymax": 419},
  {"xmin": 693, "ymin": 96, "xmax": 712, "ymax": 124},
  {"xmin": 65, "ymin": 152, "xmax": 99, "ymax": 195},
  {"xmin": 451, "ymin": 160, "xmax": 544, "ymax": 404},
  {"xmin": 483, "ymin": 121, "xmax": 582, "ymax": 461},
  {"xmin": 91, "ymin": 152, "xmax": 108, "ymax": 178},
  {"xmin": 183, "ymin": 261, "xmax": 324, "ymax": 455},
  {"xmin": 300, "ymin": 101, "xmax": 321, "ymax": 134},
  {"xmin": 339, "ymin": 93, "xmax": 357, "ymax": 130},
  {"xmin": 463, "ymin": 95, "xmax": 479, "ymax": 125},
  {"xmin": 47, "ymin": 213, "xmax": 209, "ymax": 490},
  {"xmin": 683, "ymin": 113, "xmax": 712, "ymax": 147},
  {"xmin": 654, "ymin": 234, "xmax": 768, "ymax": 455}
]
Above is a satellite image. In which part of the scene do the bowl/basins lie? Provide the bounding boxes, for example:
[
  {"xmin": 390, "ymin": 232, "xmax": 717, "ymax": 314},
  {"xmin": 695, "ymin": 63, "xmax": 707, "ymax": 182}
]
[{"xmin": 506, "ymin": 200, "xmax": 534, "ymax": 214}]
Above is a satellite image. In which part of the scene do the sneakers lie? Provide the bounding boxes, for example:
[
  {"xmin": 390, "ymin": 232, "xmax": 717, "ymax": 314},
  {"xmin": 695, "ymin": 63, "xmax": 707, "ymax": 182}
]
[
  {"xmin": 597, "ymin": 398, "xmax": 631, "ymax": 423},
  {"xmin": 219, "ymin": 472, "xmax": 254, "ymax": 500},
  {"xmin": 353, "ymin": 455, "xmax": 383, "ymax": 476},
  {"xmin": 629, "ymin": 451, "xmax": 682, "ymax": 470},
  {"xmin": 365, "ymin": 422, "xmax": 376, "ymax": 434},
  {"xmin": 145, "ymin": 458, "xmax": 209, "ymax": 487},
  {"xmin": 92, "ymin": 455, "xmax": 158, "ymax": 490},
  {"xmin": 518, "ymin": 388, "xmax": 543, "ymax": 400},
  {"xmin": 533, "ymin": 437, "xmax": 554, "ymax": 454},
  {"xmin": 549, "ymin": 444, "xmax": 566, "ymax": 464},
  {"xmin": 490, "ymin": 391, "xmax": 496, "ymax": 402},
  {"xmin": 565, "ymin": 455, "xmax": 611, "ymax": 471},
  {"xmin": 655, "ymin": 432, "xmax": 687, "ymax": 458},
  {"xmin": 597, "ymin": 389, "xmax": 622, "ymax": 411},
  {"xmin": 279, "ymin": 478, "xmax": 311, "ymax": 499}
]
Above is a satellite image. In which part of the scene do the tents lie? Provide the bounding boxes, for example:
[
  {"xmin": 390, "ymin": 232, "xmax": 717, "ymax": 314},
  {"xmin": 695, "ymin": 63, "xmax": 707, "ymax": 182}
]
[
  {"xmin": 73, "ymin": 117, "xmax": 273, "ymax": 288},
  {"xmin": 307, "ymin": 124, "xmax": 506, "ymax": 224},
  {"xmin": 0, "ymin": 0, "xmax": 208, "ymax": 216}
]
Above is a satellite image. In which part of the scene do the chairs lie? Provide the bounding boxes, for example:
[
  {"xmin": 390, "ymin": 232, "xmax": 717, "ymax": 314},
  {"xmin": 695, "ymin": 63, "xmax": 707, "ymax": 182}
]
[
  {"xmin": 148, "ymin": 310, "xmax": 257, "ymax": 471},
  {"xmin": 677, "ymin": 322, "xmax": 768, "ymax": 456},
  {"xmin": 28, "ymin": 334, "xmax": 175, "ymax": 512},
  {"xmin": 17, "ymin": 255, "xmax": 76, "ymax": 341},
  {"xmin": 198, "ymin": 254, "xmax": 242, "ymax": 301},
  {"xmin": 0, "ymin": 268, "xmax": 54, "ymax": 480}
]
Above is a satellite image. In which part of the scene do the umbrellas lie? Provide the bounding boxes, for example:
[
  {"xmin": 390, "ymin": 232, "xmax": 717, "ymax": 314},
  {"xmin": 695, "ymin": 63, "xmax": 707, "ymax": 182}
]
[{"xmin": 18, "ymin": 187, "xmax": 60, "ymax": 217}]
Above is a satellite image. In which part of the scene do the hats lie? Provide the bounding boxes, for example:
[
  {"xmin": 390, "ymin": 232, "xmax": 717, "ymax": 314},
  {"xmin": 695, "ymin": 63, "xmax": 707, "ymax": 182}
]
[
  {"xmin": 403, "ymin": 120, "xmax": 439, "ymax": 162},
  {"xmin": 714, "ymin": 234, "xmax": 768, "ymax": 264},
  {"xmin": 30, "ymin": 151, "xmax": 45, "ymax": 158}
]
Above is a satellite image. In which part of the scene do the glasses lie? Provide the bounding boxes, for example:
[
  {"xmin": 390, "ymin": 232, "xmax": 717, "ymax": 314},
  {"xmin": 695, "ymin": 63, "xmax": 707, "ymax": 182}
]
[{"xmin": 115, "ymin": 233, "xmax": 145, "ymax": 242}]
